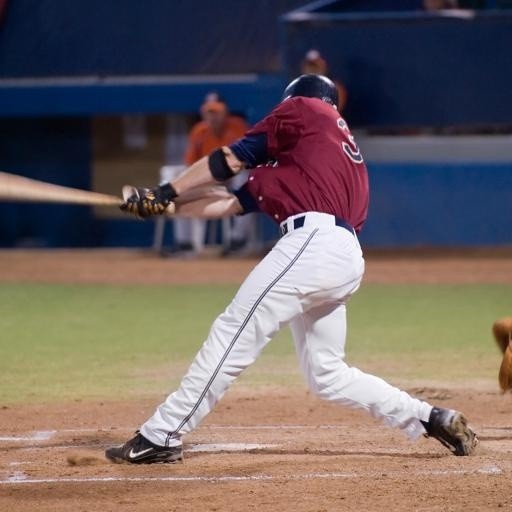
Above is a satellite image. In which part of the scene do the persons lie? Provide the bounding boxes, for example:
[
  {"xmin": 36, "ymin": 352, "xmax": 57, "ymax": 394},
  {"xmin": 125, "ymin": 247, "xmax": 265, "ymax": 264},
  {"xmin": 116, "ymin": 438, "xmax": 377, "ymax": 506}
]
[
  {"xmin": 105, "ymin": 73, "xmax": 478, "ymax": 464},
  {"xmin": 170, "ymin": 50, "xmax": 347, "ymax": 255}
]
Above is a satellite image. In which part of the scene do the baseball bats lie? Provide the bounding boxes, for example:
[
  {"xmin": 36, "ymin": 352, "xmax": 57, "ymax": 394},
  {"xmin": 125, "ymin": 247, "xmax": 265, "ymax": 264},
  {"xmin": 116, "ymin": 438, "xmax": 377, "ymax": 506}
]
[{"xmin": 0, "ymin": 170, "xmax": 176, "ymax": 219}]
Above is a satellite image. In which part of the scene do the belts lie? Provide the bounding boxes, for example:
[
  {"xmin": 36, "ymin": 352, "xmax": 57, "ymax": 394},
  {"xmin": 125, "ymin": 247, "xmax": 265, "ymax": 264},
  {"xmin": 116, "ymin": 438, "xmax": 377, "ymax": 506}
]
[{"xmin": 281, "ymin": 215, "xmax": 356, "ymax": 236}]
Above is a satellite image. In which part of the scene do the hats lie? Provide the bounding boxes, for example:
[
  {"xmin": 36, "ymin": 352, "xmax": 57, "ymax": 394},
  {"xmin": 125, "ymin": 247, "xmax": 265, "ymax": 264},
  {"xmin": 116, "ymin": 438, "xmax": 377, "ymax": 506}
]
[{"xmin": 202, "ymin": 91, "xmax": 223, "ymax": 104}]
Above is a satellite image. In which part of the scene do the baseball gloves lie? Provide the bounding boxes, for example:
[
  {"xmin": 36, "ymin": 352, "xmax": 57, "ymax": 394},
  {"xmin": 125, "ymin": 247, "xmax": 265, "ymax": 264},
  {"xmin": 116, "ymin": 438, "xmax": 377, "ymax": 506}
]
[{"xmin": 492, "ymin": 317, "xmax": 511, "ymax": 391}]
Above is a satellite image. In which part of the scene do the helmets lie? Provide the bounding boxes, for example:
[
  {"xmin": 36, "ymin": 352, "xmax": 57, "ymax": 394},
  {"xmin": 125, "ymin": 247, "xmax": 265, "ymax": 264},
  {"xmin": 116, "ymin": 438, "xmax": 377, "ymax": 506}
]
[{"xmin": 281, "ymin": 72, "xmax": 339, "ymax": 107}]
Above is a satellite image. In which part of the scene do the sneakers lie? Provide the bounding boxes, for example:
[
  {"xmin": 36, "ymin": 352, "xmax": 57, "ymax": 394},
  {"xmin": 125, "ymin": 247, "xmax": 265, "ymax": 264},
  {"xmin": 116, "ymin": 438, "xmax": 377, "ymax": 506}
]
[
  {"xmin": 421, "ymin": 406, "xmax": 480, "ymax": 457},
  {"xmin": 105, "ymin": 434, "xmax": 183, "ymax": 464}
]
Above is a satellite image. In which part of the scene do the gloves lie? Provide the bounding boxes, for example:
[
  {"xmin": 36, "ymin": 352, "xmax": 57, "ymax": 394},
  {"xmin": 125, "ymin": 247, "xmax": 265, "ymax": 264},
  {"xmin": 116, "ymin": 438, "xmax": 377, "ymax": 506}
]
[{"xmin": 120, "ymin": 183, "xmax": 178, "ymax": 223}]
